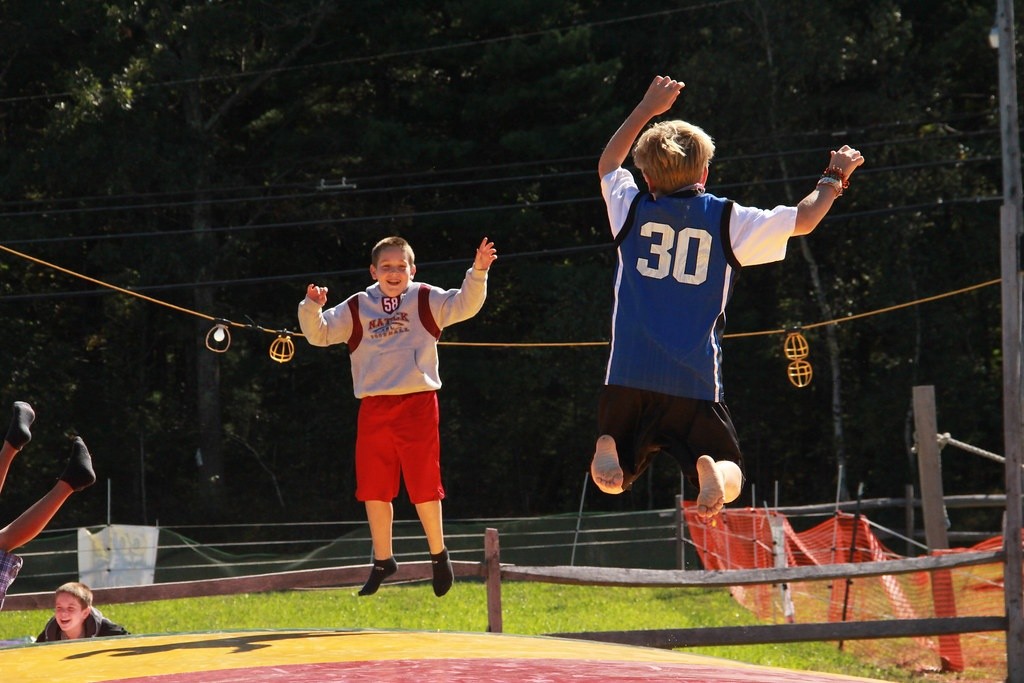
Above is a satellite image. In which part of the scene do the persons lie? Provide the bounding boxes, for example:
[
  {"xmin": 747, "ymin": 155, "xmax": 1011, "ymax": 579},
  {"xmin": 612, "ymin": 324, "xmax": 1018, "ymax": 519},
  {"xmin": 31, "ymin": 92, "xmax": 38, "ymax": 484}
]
[
  {"xmin": 590, "ymin": 75, "xmax": 865, "ymax": 518},
  {"xmin": 0, "ymin": 400, "xmax": 96, "ymax": 609},
  {"xmin": 297, "ymin": 237, "xmax": 498, "ymax": 597},
  {"xmin": 34, "ymin": 582, "xmax": 131, "ymax": 642}
]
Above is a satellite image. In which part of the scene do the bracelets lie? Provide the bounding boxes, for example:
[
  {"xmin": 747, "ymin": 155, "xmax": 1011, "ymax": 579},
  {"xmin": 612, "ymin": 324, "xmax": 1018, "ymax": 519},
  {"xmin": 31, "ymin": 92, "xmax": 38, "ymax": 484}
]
[{"xmin": 816, "ymin": 164, "xmax": 850, "ymax": 199}]
